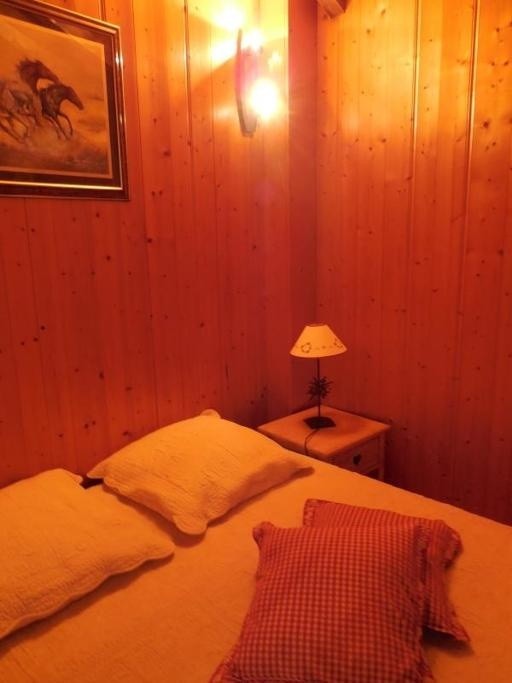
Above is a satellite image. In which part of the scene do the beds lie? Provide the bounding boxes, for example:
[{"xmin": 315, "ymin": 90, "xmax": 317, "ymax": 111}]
[{"xmin": 0, "ymin": 431, "xmax": 512, "ymax": 682}]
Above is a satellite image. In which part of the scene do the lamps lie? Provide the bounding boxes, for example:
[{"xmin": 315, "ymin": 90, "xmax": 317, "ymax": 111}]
[
  {"xmin": 287, "ymin": 320, "xmax": 348, "ymax": 431},
  {"xmin": 232, "ymin": 27, "xmax": 288, "ymax": 139}
]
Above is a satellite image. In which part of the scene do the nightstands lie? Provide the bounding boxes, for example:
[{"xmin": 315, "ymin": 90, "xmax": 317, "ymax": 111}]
[{"xmin": 262, "ymin": 403, "xmax": 392, "ymax": 482}]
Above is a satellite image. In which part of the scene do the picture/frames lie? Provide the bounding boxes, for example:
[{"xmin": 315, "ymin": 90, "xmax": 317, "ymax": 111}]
[{"xmin": 0, "ymin": 1, "xmax": 132, "ymax": 201}]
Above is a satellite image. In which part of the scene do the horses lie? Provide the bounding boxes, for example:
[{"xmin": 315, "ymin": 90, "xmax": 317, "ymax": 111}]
[{"xmin": 1, "ymin": 57, "xmax": 85, "ymax": 144}]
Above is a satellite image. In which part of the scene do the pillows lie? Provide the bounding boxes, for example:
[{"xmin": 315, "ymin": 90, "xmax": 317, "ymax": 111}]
[
  {"xmin": 84, "ymin": 408, "xmax": 312, "ymax": 539},
  {"xmin": 300, "ymin": 497, "xmax": 471, "ymax": 645},
  {"xmin": 0, "ymin": 466, "xmax": 173, "ymax": 648},
  {"xmin": 205, "ymin": 516, "xmax": 451, "ymax": 682}
]
